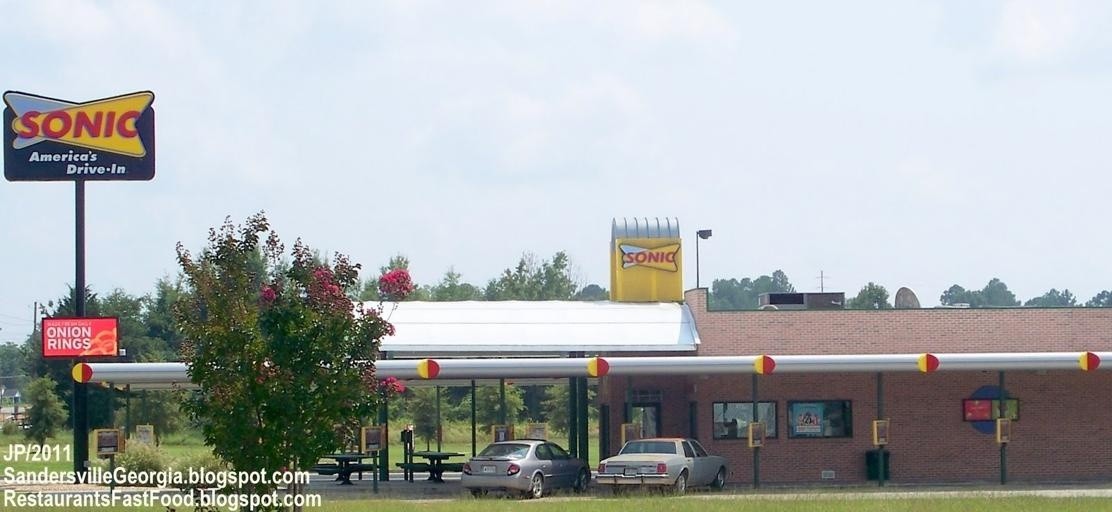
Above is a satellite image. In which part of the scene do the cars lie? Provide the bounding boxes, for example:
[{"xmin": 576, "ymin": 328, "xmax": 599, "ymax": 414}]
[
  {"xmin": 594, "ymin": 432, "xmax": 732, "ymax": 497},
  {"xmin": 461, "ymin": 436, "xmax": 594, "ymax": 500}
]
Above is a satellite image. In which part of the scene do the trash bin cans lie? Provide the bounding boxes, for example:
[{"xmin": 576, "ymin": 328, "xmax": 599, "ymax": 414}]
[{"xmin": 866, "ymin": 448, "xmax": 889, "ymax": 481}]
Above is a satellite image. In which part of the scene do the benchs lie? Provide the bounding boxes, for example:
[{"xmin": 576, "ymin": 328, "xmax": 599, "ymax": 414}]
[
  {"xmin": 312, "ymin": 453, "xmax": 379, "ymax": 485},
  {"xmin": 396, "ymin": 449, "xmax": 467, "ymax": 483}
]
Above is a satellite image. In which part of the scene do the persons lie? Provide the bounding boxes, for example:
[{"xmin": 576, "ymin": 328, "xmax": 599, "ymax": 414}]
[
  {"xmin": 799, "ymin": 412, "xmax": 818, "ymax": 426},
  {"xmin": 724, "ymin": 419, "xmax": 737, "ymax": 438}
]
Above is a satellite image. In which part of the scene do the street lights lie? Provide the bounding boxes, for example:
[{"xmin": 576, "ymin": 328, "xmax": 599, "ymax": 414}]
[{"xmin": 695, "ymin": 228, "xmax": 713, "ymax": 287}]
